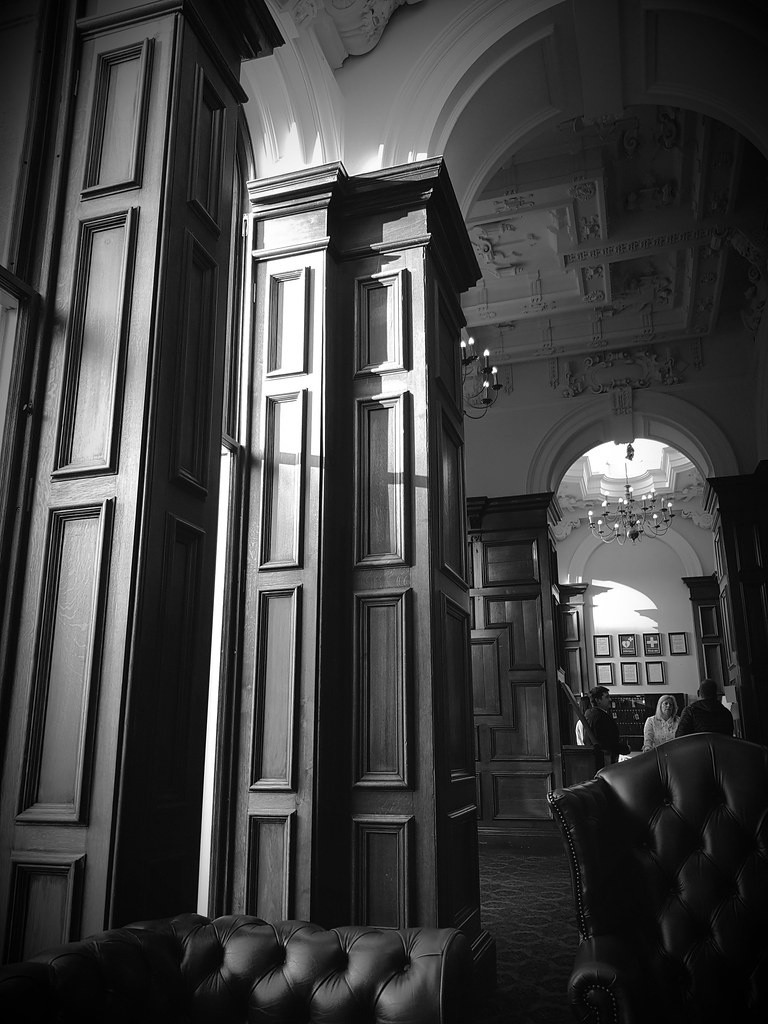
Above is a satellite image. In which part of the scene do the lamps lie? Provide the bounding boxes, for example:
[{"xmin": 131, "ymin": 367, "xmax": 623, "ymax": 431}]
[
  {"xmin": 586, "ymin": 442, "xmax": 675, "ymax": 547},
  {"xmin": 460, "ymin": 336, "xmax": 501, "ymax": 418}
]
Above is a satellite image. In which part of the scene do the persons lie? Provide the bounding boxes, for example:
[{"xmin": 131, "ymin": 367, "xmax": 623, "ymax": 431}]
[
  {"xmin": 643, "ymin": 694, "xmax": 681, "ymax": 754},
  {"xmin": 583, "ymin": 686, "xmax": 631, "ymax": 766},
  {"xmin": 576, "ymin": 696, "xmax": 592, "ymax": 746},
  {"xmin": 674, "ymin": 679, "xmax": 733, "ymax": 736}
]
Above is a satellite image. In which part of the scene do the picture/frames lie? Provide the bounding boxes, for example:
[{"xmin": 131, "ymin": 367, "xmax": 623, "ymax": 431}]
[
  {"xmin": 595, "ymin": 662, "xmax": 613, "ymax": 685},
  {"xmin": 645, "ymin": 661, "xmax": 665, "ymax": 684},
  {"xmin": 618, "ymin": 633, "xmax": 638, "ymax": 657},
  {"xmin": 667, "ymin": 631, "xmax": 688, "ymax": 655},
  {"xmin": 593, "ymin": 634, "xmax": 612, "ymax": 657},
  {"xmin": 643, "ymin": 632, "xmax": 663, "ymax": 656},
  {"xmin": 619, "ymin": 661, "xmax": 640, "ymax": 684}
]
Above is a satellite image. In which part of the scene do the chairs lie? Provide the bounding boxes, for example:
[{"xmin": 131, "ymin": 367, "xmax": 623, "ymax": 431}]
[{"xmin": 545, "ymin": 730, "xmax": 768, "ymax": 1024}]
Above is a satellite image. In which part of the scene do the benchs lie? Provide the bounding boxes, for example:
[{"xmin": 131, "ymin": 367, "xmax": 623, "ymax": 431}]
[{"xmin": 0, "ymin": 909, "xmax": 482, "ymax": 1024}]
